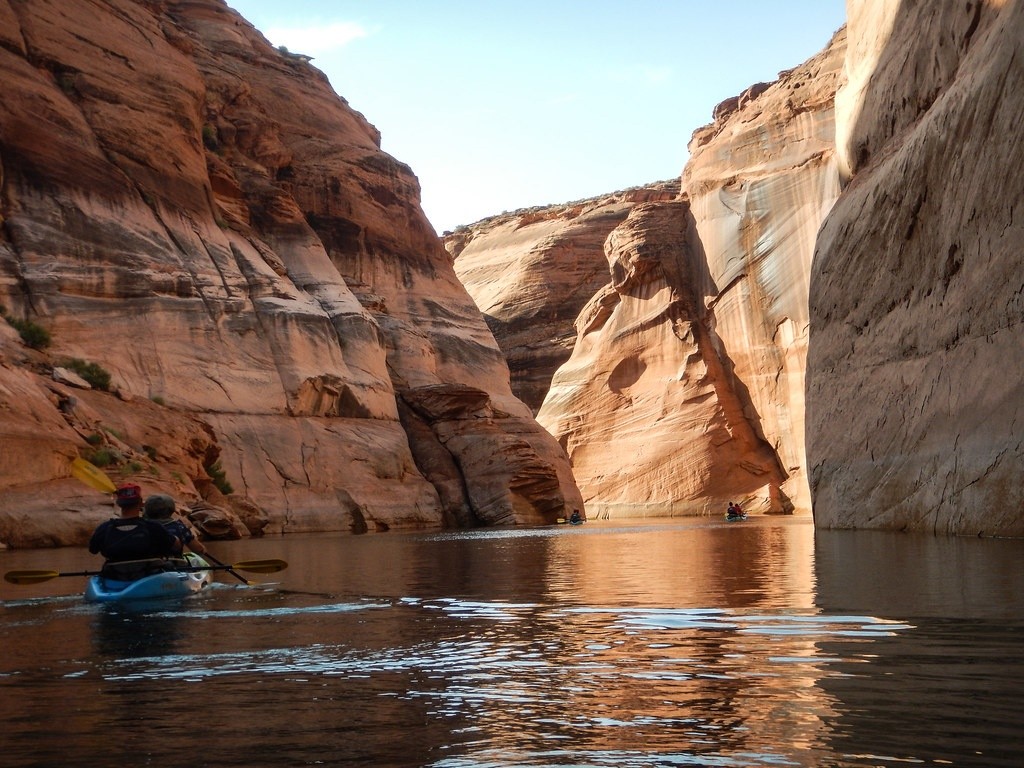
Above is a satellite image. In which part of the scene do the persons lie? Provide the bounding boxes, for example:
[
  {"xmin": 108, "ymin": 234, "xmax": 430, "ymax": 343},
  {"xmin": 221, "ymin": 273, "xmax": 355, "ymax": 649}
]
[
  {"xmin": 89, "ymin": 483, "xmax": 181, "ymax": 565},
  {"xmin": 143, "ymin": 494, "xmax": 206, "ymax": 569},
  {"xmin": 570, "ymin": 508, "xmax": 580, "ymax": 522},
  {"xmin": 727, "ymin": 502, "xmax": 745, "ymax": 518}
]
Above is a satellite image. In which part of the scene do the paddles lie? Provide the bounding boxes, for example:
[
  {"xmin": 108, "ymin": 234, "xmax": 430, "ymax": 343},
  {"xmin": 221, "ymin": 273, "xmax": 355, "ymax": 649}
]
[
  {"xmin": 69, "ymin": 459, "xmax": 257, "ymax": 585},
  {"xmin": 557, "ymin": 516, "xmax": 596, "ymax": 524},
  {"xmin": 3, "ymin": 559, "xmax": 288, "ymax": 585}
]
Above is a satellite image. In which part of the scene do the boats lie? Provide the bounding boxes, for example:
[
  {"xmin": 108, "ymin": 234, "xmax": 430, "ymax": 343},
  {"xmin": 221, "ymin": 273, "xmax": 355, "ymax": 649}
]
[
  {"xmin": 81, "ymin": 552, "xmax": 214, "ymax": 612},
  {"xmin": 726, "ymin": 515, "xmax": 749, "ymax": 522},
  {"xmin": 569, "ymin": 520, "xmax": 584, "ymax": 526}
]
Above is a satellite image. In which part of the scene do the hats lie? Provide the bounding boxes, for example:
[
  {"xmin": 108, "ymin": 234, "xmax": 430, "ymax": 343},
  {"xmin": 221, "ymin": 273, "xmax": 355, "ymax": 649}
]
[
  {"xmin": 143, "ymin": 494, "xmax": 175, "ymax": 520},
  {"xmin": 113, "ymin": 484, "xmax": 140, "ymax": 500}
]
